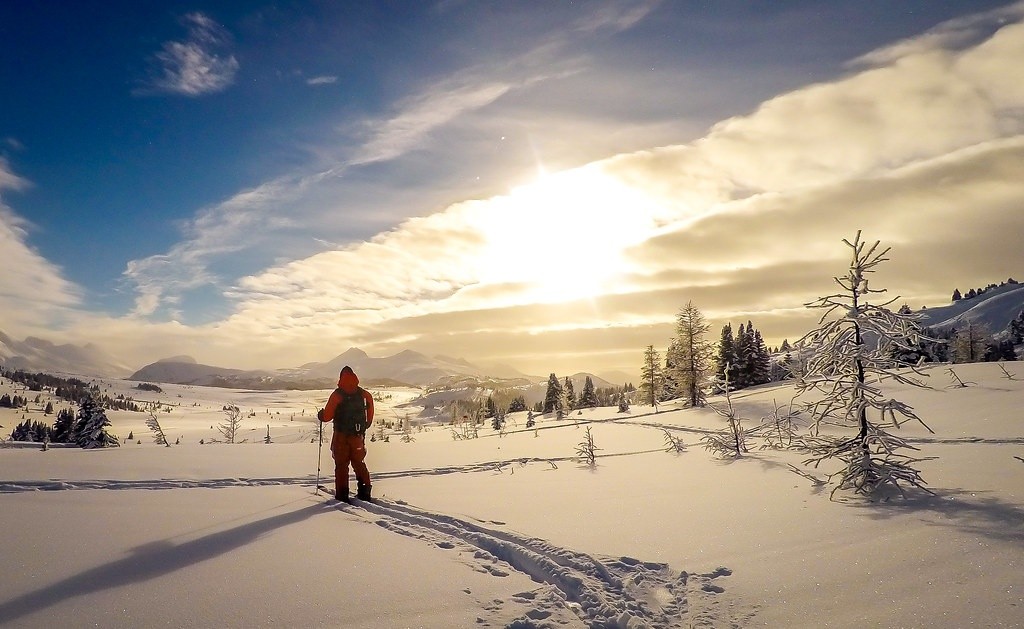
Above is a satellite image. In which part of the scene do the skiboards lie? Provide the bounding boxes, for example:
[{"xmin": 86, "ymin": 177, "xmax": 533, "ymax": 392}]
[{"xmin": 316, "ymin": 486, "xmax": 380, "ymax": 508}]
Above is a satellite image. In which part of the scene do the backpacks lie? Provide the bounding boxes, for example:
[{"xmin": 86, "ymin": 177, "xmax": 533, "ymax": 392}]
[{"xmin": 335, "ymin": 387, "xmax": 367, "ymax": 435}]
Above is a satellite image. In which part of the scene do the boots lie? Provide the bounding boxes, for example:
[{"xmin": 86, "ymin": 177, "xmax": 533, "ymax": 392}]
[
  {"xmin": 335, "ymin": 486, "xmax": 350, "ymax": 505},
  {"xmin": 354, "ymin": 482, "xmax": 372, "ymax": 503}
]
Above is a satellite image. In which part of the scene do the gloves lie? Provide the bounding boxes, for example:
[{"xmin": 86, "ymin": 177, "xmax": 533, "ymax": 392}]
[
  {"xmin": 365, "ymin": 422, "xmax": 370, "ymax": 429},
  {"xmin": 318, "ymin": 408, "xmax": 325, "ymax": 422}
]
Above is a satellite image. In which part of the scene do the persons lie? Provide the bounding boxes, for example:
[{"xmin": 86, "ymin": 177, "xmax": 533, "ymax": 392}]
[{"xmin": 317, "ymin": 366, "xmax": 374, "ymax": 503}]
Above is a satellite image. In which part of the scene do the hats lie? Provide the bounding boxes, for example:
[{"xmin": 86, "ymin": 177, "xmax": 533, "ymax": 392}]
[{"xmin": 340, "ymin": 366, "xmax": 354, "ymax": 377}]
